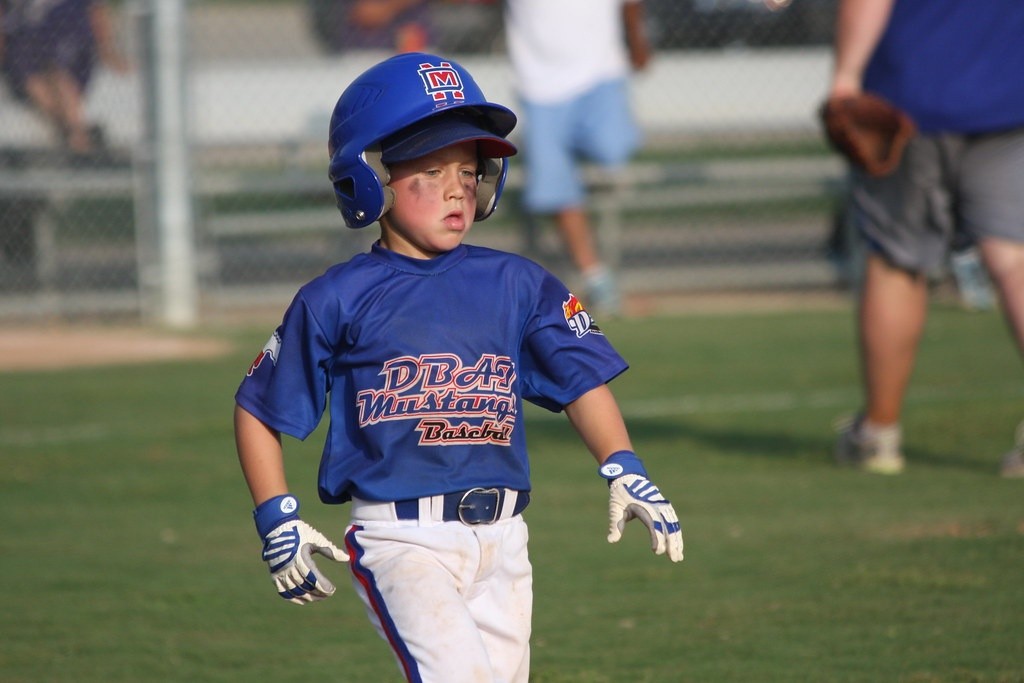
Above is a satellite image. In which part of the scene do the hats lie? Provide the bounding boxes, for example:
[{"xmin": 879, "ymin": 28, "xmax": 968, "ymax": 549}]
[{"xmin": 364, "ymin": 106, "xmax": 518, "ymax": 166}]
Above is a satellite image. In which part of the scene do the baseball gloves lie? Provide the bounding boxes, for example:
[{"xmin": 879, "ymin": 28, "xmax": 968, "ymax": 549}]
[{"xmin": 821, "ymin": 93, "xmax": 915, "ymax": 179}]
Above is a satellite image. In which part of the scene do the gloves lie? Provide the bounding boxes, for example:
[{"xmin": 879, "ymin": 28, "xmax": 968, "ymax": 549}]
[
  {"xmin": 252, "ymin": 494, "xmax": 349, "ymax": 604},
  {"xmin": 598, "ymin": 450, "xmax": 685, "ymax": 563}
]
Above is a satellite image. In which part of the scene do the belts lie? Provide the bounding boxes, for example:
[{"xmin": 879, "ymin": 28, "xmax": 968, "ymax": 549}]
[{"xmin": 395, "ymin": 491, "xmax": 534, "ymax": 527}]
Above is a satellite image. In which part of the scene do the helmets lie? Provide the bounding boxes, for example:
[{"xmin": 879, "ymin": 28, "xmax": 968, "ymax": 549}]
[{"xmin": 327, "ymin": 53, "xmax": 516, "ymax": 230}]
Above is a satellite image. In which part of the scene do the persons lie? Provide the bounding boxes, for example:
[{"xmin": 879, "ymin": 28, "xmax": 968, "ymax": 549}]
[
  {"xmin": 0, "ymin": 0, "xmax": 134, "ymax": 168},
  {"xmin": 819, "ymin": 0, "xmax": 1024, "ymax": 481},
  {"xmin": 233, "ymin": 51, "xmax": 683, "ymax": 683},
  {"xmin": 498, "ymin": 0, "xmax": 652, "ymax": 319}
]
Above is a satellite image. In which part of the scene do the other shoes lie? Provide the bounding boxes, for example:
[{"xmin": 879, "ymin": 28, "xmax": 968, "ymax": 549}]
[
  {"xmin": 843, "ymin": 415, "xmax": 906, "ymax": 477},
  {"xmin": 1000, "ymin": 425, "xmax": 1024, "ymax": 480}
]
